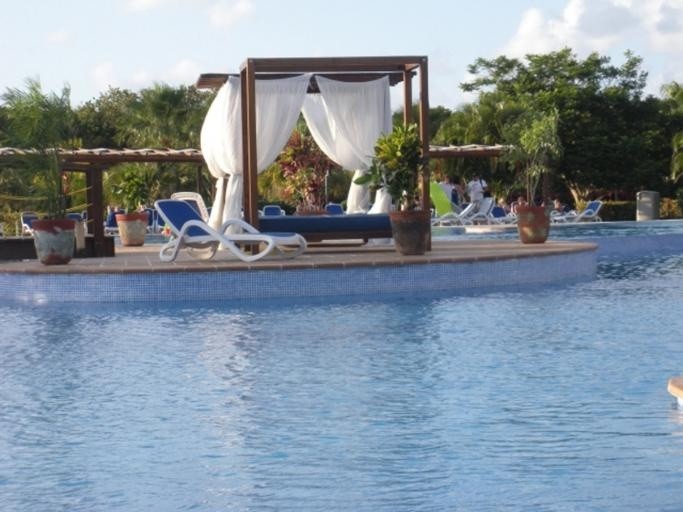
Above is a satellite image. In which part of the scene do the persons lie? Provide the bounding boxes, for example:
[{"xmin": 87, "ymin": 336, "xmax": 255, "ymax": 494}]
[
  {"xmin": 498, "ymin": 196, "xmax": 570, "ymax": 215},
  {"xmin": 439, "ymin": 172, "xmax": 488, "ymax": 207}
]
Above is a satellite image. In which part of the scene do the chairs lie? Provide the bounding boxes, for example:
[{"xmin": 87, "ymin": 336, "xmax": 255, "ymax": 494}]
[
  {"xmin": 395, "ymin": 196, "xmax": 603, "ymax": 229},
  {"xmin": 23, "ymin": 190, "xmax": 345, "ymax": 263}
]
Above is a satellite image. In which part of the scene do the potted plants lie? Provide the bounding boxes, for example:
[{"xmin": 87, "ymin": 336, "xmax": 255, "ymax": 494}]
[
  {"xmin": 507, "ymin": 112, "xmax": 574, "ymax": 243},
  {"xmin": 1, "ymin": 77, "xmax": 79, "ymax": 265},
  {"xmin": 113, "ymin": 157, "xmax": 156, "ymax": 246},
  {"xmin": 353, "ymin": 122, "xmax": 433, "ymax": 258}
]
